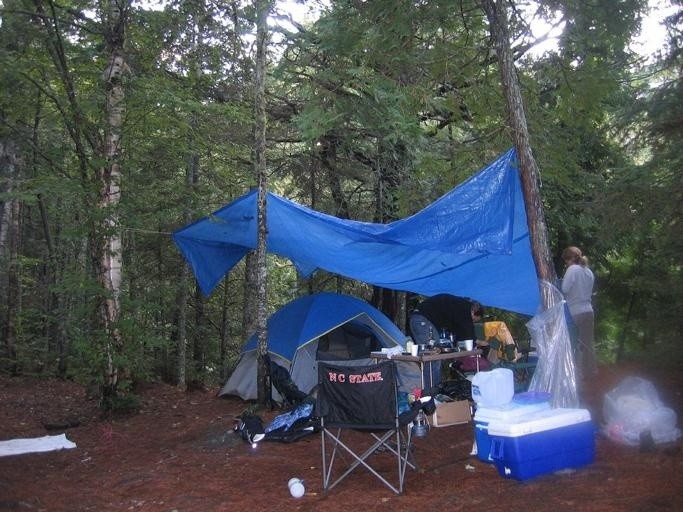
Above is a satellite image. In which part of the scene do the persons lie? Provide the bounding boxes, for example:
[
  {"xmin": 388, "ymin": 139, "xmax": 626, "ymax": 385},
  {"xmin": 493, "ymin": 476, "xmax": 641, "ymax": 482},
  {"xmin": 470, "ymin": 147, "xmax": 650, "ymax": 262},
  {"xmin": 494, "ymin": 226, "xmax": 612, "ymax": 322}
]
[
  {"xmin": 562, "ymin": 246, "xmax": 596, "ymax": 380},
  {"xmin": 409, "ymin": 293, "xmax": 483, "ymax": 394}
]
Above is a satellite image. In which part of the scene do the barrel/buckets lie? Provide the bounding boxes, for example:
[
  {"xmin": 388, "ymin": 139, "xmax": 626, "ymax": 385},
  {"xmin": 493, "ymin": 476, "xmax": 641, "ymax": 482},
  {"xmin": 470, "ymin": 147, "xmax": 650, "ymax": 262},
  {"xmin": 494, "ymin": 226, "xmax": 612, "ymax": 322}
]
[{"xmin": 471, "ymin": 367, "xmax": 515, "ymax": 408}]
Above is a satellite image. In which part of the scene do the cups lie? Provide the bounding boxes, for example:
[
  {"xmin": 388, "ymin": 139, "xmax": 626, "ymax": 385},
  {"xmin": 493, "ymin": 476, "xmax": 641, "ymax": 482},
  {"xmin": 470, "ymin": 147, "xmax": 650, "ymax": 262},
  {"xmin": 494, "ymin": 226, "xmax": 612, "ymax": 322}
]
[
  {"xmin": 464, "ymin": 339, "xmax": 473, "ymax": 351},
  {"xmin": 410, "ymin": 344, "xmax": 418, "ymax": 356}
]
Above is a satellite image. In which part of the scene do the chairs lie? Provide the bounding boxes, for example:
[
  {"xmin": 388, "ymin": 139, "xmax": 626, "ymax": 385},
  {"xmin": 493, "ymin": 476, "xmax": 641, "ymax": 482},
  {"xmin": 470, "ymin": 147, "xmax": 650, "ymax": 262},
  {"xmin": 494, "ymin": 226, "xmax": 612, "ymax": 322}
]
[{"xmin": 312, "ymin": 357, "xmax": 425, "ymax": 498}]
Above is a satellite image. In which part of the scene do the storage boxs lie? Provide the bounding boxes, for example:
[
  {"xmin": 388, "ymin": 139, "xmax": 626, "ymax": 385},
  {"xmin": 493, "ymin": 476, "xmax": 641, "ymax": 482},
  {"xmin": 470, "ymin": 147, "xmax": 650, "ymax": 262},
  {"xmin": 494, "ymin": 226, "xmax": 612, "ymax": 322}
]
[
  {"xmin": 422, "ymin": 400, "xmax": 471, "ymax": 428},
  {"xmin": 470, "ymin": 399, "xmax": 596, "ymax": 482}
]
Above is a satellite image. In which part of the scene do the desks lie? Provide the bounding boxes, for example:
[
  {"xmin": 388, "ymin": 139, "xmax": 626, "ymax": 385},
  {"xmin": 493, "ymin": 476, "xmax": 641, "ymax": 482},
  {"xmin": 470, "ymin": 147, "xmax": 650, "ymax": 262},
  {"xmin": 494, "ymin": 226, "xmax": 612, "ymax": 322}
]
[{"xmin": 372, "ymin": 348, "xmax": 485, "ymax": 405}]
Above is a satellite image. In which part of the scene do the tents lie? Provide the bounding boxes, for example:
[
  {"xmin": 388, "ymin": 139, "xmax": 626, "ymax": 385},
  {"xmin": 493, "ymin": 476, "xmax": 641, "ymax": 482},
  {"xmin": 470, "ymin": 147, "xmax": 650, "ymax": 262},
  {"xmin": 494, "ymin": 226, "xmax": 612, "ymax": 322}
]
[{"xmin": 217, "ymin": 292, "xmax": 422, "ymax": 409}]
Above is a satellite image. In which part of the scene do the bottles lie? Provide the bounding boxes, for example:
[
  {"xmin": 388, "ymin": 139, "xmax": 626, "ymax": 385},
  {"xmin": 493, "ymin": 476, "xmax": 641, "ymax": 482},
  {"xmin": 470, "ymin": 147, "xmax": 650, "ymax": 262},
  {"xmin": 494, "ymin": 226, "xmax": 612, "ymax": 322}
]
[
  {"xmin": 412, "ymin": 410, "xmax": 427, "ymax": 438},
  {"xmin": 286, "ymin": 477, "xmax": 305, "ymax": 498},
  {"xmin": 428, "ymin": 325, "xmax": 435, "ymax": 348}
]
